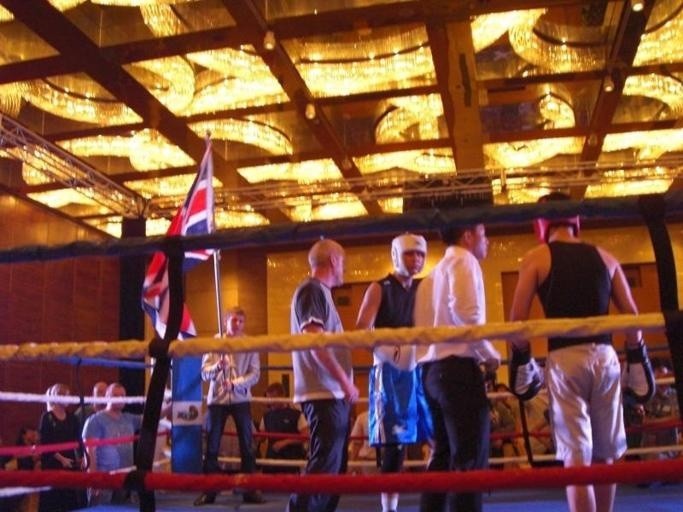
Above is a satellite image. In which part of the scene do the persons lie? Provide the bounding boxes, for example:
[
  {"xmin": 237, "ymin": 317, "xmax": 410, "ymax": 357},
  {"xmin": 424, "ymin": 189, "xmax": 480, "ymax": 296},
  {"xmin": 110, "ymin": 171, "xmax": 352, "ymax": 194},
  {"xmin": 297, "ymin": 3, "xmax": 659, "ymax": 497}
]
[
  {"xmin": 352, "ymin": 232, "xmax": 437, "ymax": 512},
  {"xmin": 508, "ymin": 192, "xmax": 655, "ymax": 511},
  {"xmin": 483, "ymin": 374, "xmax": 561, "ymax": 469},
  {"xmin": 255, "ymin": 384, "xmax": 309, "ymax": 476},
  {"xmin": 289, "ymin": 237, "xmax": 361, "ymax": 512},
  {"xmin": 0, "ymin": 381, "xmax": 173, "ymax": 512},
  {"xmin": 193, "ymin": 308, "xmax": 264, "ymax": 506},
  {"xmin": 620, "ymin": 367, "xmax": 682, "ymax": 463},
  {"xmin": 354, "ymin": 411, "xmax": 381, "ymax": 473},
  {"xmin": 409, "ymin": 215, "xmax": 502, "ymax": 512}
]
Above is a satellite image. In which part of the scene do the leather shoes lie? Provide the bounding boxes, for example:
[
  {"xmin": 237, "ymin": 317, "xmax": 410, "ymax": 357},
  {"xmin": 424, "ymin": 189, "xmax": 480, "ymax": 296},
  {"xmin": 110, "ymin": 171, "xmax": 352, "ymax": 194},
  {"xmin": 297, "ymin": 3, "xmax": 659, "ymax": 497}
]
[{"xmin": 192, "ymin": 490, "xmax": 270, "ymax": 505}]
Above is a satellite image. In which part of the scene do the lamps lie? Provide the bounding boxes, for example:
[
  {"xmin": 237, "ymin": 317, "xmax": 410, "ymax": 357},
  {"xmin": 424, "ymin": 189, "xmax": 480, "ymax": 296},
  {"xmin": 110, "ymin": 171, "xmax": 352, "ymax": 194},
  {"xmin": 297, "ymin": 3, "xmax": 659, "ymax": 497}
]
[{"xmin": 0, "ymin": 0, "xmax": 683, "ymax": 237}]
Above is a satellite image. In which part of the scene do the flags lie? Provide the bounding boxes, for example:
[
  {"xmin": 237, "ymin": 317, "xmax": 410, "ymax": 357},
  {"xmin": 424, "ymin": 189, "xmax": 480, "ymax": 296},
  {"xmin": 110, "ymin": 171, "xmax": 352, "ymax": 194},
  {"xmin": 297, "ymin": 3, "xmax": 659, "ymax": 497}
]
[{"xmin": 141, "ymin": 143, "xmax": 215, "ymax": 340}]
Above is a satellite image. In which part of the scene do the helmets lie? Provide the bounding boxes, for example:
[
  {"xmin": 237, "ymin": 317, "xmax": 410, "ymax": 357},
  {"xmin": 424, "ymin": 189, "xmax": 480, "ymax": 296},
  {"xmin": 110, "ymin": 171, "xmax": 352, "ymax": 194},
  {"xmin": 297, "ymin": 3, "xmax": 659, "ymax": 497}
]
[
  {"xmin": 389, "ymin": 231, "xmax": 428, "ymax": 279},
  {"xmin": 533, "ymin": 190, "xmax": 581, "ymax": 244}
]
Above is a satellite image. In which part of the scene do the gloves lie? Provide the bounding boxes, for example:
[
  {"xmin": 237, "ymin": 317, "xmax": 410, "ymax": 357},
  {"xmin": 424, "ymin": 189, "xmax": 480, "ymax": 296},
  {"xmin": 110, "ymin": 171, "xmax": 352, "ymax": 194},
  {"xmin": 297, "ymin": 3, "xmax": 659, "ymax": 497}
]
[
  {"xmin": 506, "ymin": 339, "xmax": 546, "ymax": 402},
  {"xmin": 620, "ymin": 336, "xmax": 657, "ymax": 404}
]
